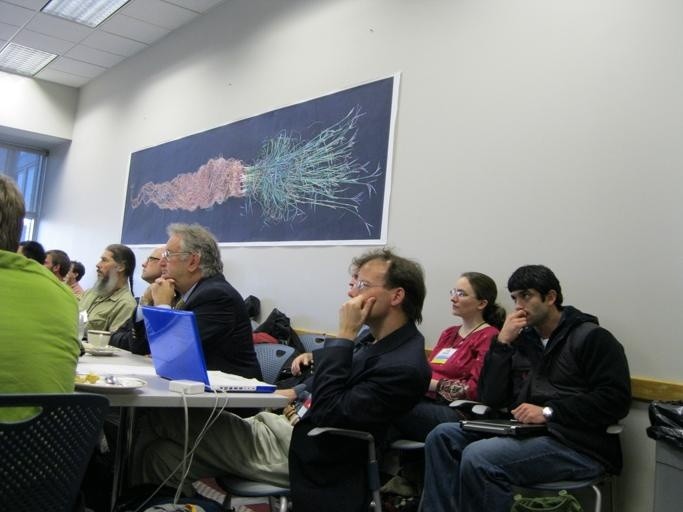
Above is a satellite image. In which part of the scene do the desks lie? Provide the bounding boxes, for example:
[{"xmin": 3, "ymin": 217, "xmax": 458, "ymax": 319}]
[{"xmin": 74, "ymin": 340, "xmax": 290, "ymax": 510}]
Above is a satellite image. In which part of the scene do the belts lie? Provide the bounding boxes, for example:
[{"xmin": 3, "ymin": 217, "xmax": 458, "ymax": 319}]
[{"xmin": 285, "ymin": 405, "xmax": 301, "ymax": 426}]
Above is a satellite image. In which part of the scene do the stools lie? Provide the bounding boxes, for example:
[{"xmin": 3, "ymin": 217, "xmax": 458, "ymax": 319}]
[
  {"xmin": 394, "ymin": 438, "xmax": 424, "ymax": 453},
  {"xmin": 510, "ymin": 479, "xmax": 606, "ymax": 511}
]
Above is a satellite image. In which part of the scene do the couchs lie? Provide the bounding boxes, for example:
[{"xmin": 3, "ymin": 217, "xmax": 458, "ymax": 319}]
[{"xmin": 286, "ymin": 328, "xmax": 442, "ymax": 359}]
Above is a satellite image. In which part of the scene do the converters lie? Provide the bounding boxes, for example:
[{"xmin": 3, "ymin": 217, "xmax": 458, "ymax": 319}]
[{"xmin": 166, "ymin": 376, "xmax": 206, "ymax": 395}]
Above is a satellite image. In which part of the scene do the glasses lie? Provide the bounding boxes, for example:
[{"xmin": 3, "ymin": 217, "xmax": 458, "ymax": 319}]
[
  {"xmin": 160, "ymin": 250, "xmax": 193, "ymax": 258},
  {"xmin": 146, "ymin": 256, "xmax": 160, "ymax": 263},
  {"xmin": 449, "ymin": 289, "xmax": 485, "ymax": 301},
  {"xmin": 355, "ymin": 281, "xmax": 398, "ymax": 290}
]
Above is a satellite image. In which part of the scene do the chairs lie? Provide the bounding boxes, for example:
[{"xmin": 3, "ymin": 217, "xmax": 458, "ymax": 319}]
[
  {"xmin": 0, "ymin": 393, "xmax": 112, "ymax": 512},
  {"xmin": 215, "ymin": 477, "xmax": 291, "ymax": 512}
]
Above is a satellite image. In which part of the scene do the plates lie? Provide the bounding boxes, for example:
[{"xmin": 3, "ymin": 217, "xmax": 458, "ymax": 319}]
[
  {"xmin": 75, "ymin": 376, "xmax": 147, "ymax": 393},
  {"xmin": 84, "ymin": 348, "xmax": 121, "ymax": 355}
]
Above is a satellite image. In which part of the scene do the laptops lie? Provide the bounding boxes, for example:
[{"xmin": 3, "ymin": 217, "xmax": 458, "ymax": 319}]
[
  {"xmin": 457, "ymin": 418, "xmax": 546, "ymax": 440},
  {"xmin": 138, "ymin": 302, "xmax": 278, "ymax": 395}
]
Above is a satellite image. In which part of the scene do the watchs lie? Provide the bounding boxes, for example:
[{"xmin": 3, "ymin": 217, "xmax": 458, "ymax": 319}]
[{"xmin": 539, "ymin": 406, "xmax": 554, "ymax": 422}]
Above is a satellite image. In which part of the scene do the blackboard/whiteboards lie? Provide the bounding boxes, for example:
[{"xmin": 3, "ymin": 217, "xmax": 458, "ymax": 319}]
[{"xmin": 117, "ymin": 71, "xmax": 401, "ymax": 249}]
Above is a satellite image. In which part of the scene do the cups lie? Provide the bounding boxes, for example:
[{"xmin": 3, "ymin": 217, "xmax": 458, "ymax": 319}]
[{"xmin": 87, "ymin": 331, "xmax": 111, "ymax": 347}]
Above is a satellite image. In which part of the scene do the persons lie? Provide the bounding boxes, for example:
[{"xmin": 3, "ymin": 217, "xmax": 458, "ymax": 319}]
[
  {"xmin": 271, "ymin": 259, "xmax": 360, "ymax": 411},
  {"xmin": 130, "ymin": 248, "xmax": 430, "ymax": 491},
  {"xmin": 129, "ymin": 221, "xmax": 262, "ymax": 382},
  {"xmin": 375, "ymin": 271, "xmax": 506, "ymax": 496},
  {"xmin": 43, "ymin": 250, "xmax": 70, "ymax": 278},
  {"xmin": 421, "ymin": 265, "xmax": 632, "ymax": 511},
  {"xmin": 109, "ymin": 246, "xmax": 168, "ymax": 359},
  {"xmin": 77, "ymin": 244, "xmax": 138, "ymax": 330},
  {"xmin": 63, "ymin": 261, "xmax": 85, "ymax": 297},
  {"xmin": 18, "ymin": 241, "xmax": 45, "ymax": 263},
  {"xmin": 0, "ymin": 175, "xmax": 82, "ymax": 424}
]
